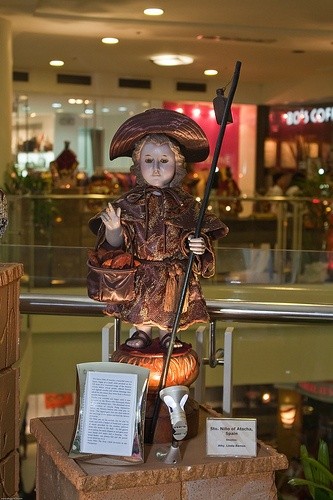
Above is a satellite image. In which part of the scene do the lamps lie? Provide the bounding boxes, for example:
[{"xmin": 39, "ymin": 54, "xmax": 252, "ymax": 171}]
[{"xmin": 159, "ymin": 385, "xmax": 190, "ymax": 465}]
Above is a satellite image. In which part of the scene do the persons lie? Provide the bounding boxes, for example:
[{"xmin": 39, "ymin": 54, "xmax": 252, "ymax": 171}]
[
  {"xmin": 88, "ymin": 108, "xmax": 229, "ymax": 351},
  {"xmin": 266, "ymin": 172, "xmax": 304, "ymax": 215},
  {"xmin": 51, "ymin": 141, "xmax": 79, "ymax": 177},
  {"xmin": 210, "ymin": 167, "xmax": 242, "ymax": 222}
]
